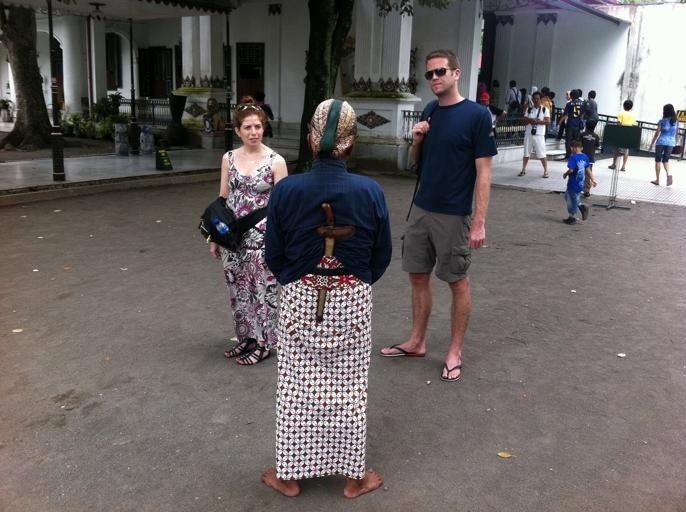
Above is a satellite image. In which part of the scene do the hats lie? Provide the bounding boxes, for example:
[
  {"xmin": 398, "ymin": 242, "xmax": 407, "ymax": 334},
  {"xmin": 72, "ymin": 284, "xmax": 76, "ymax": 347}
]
[
  {"xmin": 480, "ymin": 92, "xmax": 490, "ymax": 101},
  {"xmin": 309, "ymin": 99, "xmax": 357, "ymax": 159}
]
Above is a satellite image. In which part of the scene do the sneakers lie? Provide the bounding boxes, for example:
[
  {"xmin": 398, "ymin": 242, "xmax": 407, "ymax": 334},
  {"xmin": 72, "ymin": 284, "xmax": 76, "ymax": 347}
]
[
  {"xmin": 620, "ymin": 167, "xmax": 626, "ymax": 171},
  {"xmin": 651, "ymin": 181, "xmax": 659, "ymax": 185},
  {"xmin": 579, "ymin": 204, "xmax": 589, "ymax": 221},
  {"xmin": 667, "ymin": 175, "xmax": 672, "ymax": 186},
  {"xmin": 520, "ymin": 170, "xmax": 528, "ymax": 176},
  {"xmin": 608, "ymin": 164, "xmax": 616, "ymax": 169},
  {"xmin": 563, "ymin": 216, "xmax": 577, "ymax": 225},
  {"xmin": 543, "ymin": 172, "xmax": 549, "ymax": 178}
]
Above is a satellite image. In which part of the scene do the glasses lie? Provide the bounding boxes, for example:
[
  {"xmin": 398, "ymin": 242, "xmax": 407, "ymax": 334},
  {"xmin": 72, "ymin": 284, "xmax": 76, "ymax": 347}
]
[
  {"xmin": 234, "ymin": 103, "xmax": 262, "ymax": 113},
  {"xmin": 424, "ymin": 67, "xmax": 457, "ymax": 80}
]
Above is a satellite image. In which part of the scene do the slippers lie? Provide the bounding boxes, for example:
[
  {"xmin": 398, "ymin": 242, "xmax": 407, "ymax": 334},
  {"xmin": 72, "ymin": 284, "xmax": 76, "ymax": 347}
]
[
  {"xmin": 440, "ymin": 350, "xmax": 462, "ymax": 382},
  {"xmin": 379, "ymin": 344, "xmax": 425, "ymax": 357}
]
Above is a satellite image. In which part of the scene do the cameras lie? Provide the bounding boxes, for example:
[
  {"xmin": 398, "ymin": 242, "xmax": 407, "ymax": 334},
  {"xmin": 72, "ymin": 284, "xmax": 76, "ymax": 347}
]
[{"xmin": 529, "ymin": 126, "xmax": 538, "ymax": 135}]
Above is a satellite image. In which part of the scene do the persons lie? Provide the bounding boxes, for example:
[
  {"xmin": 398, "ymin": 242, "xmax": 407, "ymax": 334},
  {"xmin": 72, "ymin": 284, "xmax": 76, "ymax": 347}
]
[
  {"xmin": 581, "ymin": 90, "xmax": 598, "ymax": 150},
  {"xmin": 608, "ymin": 99, "xmax": 639, "ymax": 172},
  {"xmin": 382, "ymin": 47, "xmax": 500, "ymax": 383},
  {"xmin": 556, "ymin": 89, "xmax": 586, "ymax": 162},
  {"xmin": 478, "ymin": 91, "xmax": 506, "ymax": 141},
  {"xmin": 540, "ymin": 86, "xmax": 553, "ymax": 142},
  {"xmin": 208, "ymin": 95, "xmax": 290, "ymax": 366},
  {"xmin": 580, "ymin": 118, "xmax": 600, "ymax": 197},
  {"xmin": 517, "ymin": 91, "xmax": 550, "ymax": 178},
  {"xmin": 522, "ymin": 85, "xmax": 538, "ymax": 118},
  {"xmin": 577, "ymin": 89, "xmax": 585, "ymax": 102},
  {"xmin": 490, "ymin": 79, "xmax": 500, "ymax": 108},
  {"xmin": 520, "ymin": 88, "xmax": 529, "ymax": 114},
  {"xmin": 562, "ymin": 139, "xmax": 598, "ymax": 224},
  {"xmin": 647, "ymin": 104, "xmax": 680, "ymax": 186},
  {"xmin": 260, "ymin": 96, "xmax": 393, "ymax": 499},
  {"xmin": 503, "ymin": 80, "xmax": 522, "ymax": 139},
  {"xmin": 476, "ymin": 74, "xmax": 490, "ymax": 104},
  {"xmin": 555, "ymin": 90, "xmax": 573, "ymax": 142},
  {"xmin": 548, "ymin": 91, "xmax": 556, "ymax": 122}
]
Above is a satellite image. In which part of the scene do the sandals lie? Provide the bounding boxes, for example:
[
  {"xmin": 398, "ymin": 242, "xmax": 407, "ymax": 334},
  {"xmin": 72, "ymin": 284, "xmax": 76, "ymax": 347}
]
[
  {"xmin": 223, "ymin": 338, "xmax": 257, "ymax": 358},
  {"xmin": 236, "ymin": 343, "xmax": 271, "ymax": 365}
]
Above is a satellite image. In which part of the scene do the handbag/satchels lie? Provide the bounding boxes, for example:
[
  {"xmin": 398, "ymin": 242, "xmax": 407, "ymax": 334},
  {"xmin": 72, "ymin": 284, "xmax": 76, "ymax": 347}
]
[
  {"xmin": 579, "ymin": 120, "xmax": 584, "ymax": 130},
  {"xmin": 198, "ymin": 196, "xmax": 243, "ymax": 253}
]
